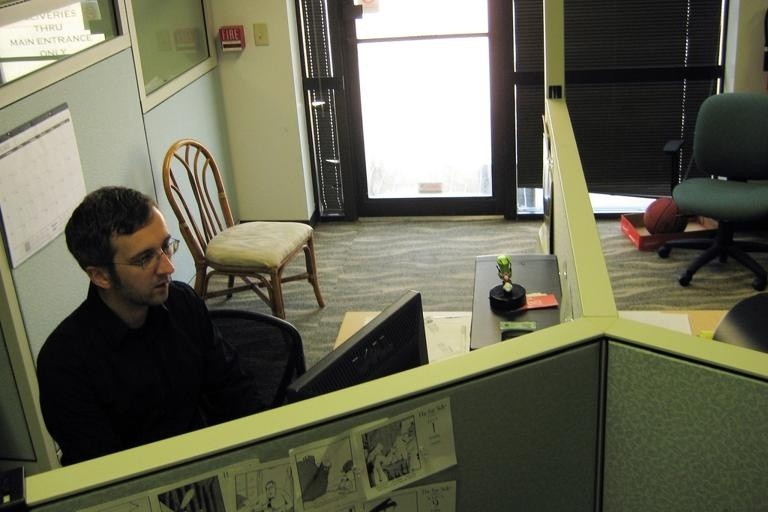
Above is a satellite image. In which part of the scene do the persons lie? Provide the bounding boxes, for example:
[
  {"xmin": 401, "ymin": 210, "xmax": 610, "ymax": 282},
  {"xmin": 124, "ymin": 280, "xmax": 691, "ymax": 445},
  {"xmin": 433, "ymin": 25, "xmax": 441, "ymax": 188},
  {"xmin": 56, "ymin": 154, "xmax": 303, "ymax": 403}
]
[{"xmin": 34, "ymin": 185, "xmax": 245, "ymax": 469}]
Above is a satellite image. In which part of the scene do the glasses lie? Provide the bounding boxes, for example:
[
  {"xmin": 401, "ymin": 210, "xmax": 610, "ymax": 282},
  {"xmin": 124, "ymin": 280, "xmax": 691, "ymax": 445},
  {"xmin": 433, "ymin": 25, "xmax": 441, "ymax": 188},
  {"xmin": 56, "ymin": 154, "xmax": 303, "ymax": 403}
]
[{"xmin": 112, "ymin": 238, "xmax": 179, "ymax": 269}]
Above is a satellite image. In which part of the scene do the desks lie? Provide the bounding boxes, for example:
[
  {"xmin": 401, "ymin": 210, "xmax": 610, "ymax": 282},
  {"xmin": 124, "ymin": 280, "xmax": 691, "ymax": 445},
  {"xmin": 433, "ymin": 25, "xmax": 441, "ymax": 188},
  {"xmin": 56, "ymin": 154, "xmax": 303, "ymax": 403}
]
[
  {"xmin": 620, "ymin": 309, "xmax": 727, "ymax": 339},
  {"xmin": 333, "ymin": 312, "xmax": 472, "ymax": 365}
]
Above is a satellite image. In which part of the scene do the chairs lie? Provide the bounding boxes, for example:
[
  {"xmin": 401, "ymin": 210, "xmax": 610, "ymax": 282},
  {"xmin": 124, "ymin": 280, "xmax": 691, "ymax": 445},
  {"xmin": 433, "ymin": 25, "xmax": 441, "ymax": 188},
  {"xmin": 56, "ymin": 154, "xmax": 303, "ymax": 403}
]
[
  {"xmin": 657, "ymin": 92, "xmax": 768, "ymax": 291},
  {"xmin": 163, "ymin": 140, "xmax": 325, "ymax": 318}
]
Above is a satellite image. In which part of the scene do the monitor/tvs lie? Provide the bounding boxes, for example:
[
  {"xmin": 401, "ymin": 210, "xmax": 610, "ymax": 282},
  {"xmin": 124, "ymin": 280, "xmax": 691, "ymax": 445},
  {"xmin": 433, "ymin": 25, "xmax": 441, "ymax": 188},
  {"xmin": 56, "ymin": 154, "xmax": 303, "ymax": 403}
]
[{"xmin": 280, "ymin": 290, "xmax": 430, "ymax": 404}]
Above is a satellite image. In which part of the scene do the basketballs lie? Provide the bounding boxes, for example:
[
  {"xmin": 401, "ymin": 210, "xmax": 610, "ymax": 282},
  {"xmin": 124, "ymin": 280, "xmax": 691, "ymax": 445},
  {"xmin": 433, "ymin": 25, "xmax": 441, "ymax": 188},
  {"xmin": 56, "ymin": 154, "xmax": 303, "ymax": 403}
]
[{"xmin": 643, "ymin": 197, "xmax": 688, "ymax": 235}]
